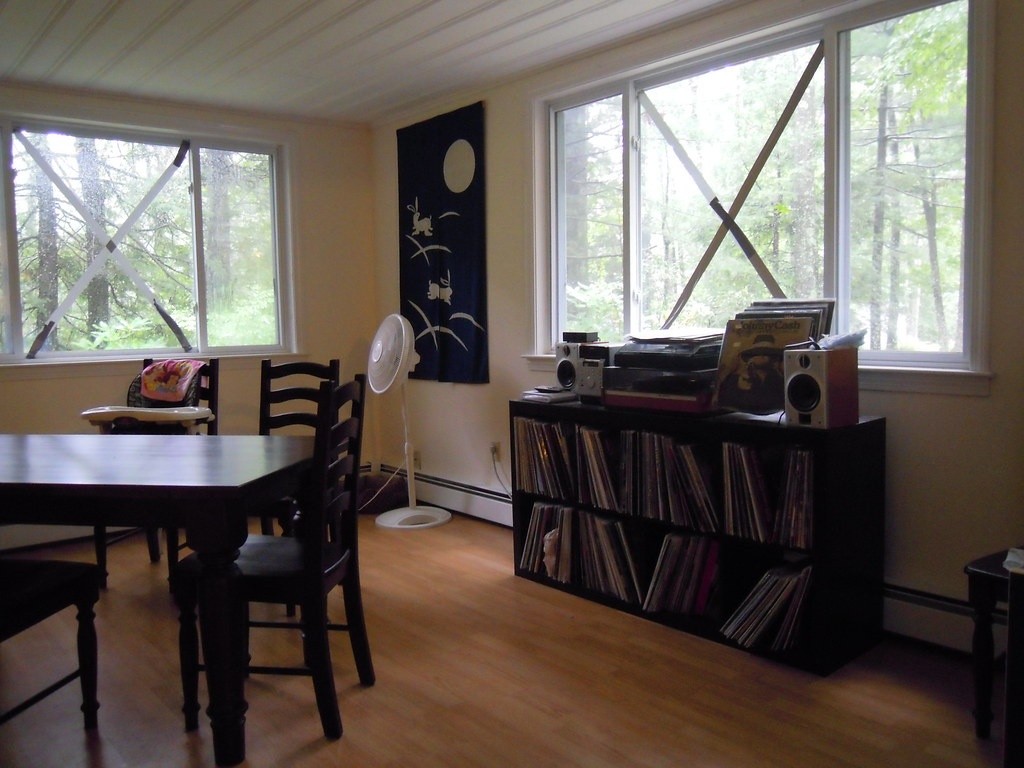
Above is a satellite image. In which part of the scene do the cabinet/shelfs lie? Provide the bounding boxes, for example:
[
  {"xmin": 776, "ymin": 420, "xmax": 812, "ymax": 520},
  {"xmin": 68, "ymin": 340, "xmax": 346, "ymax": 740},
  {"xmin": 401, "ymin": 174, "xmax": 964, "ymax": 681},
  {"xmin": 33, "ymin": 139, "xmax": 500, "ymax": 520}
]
[{"xmin": 509, "ymin": 397, "xmax": 888, "ymax": 677}]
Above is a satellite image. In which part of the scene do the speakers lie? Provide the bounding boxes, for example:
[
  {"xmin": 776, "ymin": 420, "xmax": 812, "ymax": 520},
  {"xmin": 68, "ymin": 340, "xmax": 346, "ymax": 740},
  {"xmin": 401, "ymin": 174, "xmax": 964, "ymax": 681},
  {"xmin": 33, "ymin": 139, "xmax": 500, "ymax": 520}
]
[
  {"xmin": 554, "ymin": 342, "xmax": 609, "ymax": 393},
  {"xmin": 783, "ymin": 347, "xmax": 860, "ymax": 430}
]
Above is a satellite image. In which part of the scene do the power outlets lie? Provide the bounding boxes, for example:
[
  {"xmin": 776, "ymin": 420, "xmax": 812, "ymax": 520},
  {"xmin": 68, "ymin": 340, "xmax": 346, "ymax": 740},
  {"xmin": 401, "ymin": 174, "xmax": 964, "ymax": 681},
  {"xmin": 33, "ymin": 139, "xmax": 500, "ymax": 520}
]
[
  {"xmin": 414, "ymin": 450, "xmax": 422, "ymax": 470},
  {"xmin": 490, "ymin": 441, "xmax": 501, "ymax": 462}
]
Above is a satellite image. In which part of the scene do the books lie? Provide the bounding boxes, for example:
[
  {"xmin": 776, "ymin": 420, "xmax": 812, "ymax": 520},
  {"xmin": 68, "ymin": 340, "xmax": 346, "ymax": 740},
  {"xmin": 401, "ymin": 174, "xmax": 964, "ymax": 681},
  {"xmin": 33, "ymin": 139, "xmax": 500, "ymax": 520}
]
[
  {"xmin": 522, "ymin": 389, "xmax": 577, "ymax": 404},
  {"xmin": 710, "ymin": 301, "xmax": 835, "ymax": 417},
  {"xmin": 511, "ymin": 416, "xmax": 577, "ymax": 499},
  {"xmin": 771, "ymin": 443, "xmax": 813, "ymax": 549},
  {"xmin": 642, "ymin": 531, "xmax": 721, "ymax": 616},
  {"xmin": 578, "ymin": 511, "xmax": 660, "ymax": 608},
  {"xmin": 574, "ymin": 424, "xmax": 719, "ymax": 532},
  {"xmin": 520, "ymin": 501, "xmax": 573, "ymax": 585},
  {"xmin": 719, "ymin": 562, "xmax": 812, "ymax": 651},
  {"xmin": 722, "ymin": 443, "xmax": 774, "ymax": 542}
]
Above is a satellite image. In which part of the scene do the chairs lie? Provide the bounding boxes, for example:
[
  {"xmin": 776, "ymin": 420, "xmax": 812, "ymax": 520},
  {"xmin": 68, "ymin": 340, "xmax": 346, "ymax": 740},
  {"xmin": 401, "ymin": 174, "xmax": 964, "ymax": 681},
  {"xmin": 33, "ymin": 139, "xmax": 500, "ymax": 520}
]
[
  {"xmin": 166, "ymin": 359, "xmax": 340, "ymax": 618},
  {"xmin": 94, "ymin": 357, "xmax": 220, "ymax": 594},
  {"xmin": 0, "ymin": 556, "xmax": 109, "ymax": 730},
  {"xmin": 167, "ymin": 374, "xmax": 376, "ymax": 740}
]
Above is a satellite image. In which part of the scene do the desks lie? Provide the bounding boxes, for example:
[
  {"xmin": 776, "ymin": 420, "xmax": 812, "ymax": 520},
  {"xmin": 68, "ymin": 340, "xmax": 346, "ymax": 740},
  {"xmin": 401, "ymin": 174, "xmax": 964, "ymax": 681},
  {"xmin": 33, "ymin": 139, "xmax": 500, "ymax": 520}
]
[
  {"xmin": 0, "ymin": 434, "xmax": 316, "ymax": 768},
  {"xmin": 964, "ymin": 546, "xmax": 1024, "ymax": 741}
]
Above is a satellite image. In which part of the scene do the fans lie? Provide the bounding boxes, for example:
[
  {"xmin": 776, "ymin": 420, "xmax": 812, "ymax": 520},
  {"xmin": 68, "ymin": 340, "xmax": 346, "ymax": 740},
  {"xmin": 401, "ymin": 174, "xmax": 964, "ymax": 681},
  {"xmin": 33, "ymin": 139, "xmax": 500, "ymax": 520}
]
[{"xmin": 367, "ymin": 313, "xmax": 452, "ymax": 530}]
